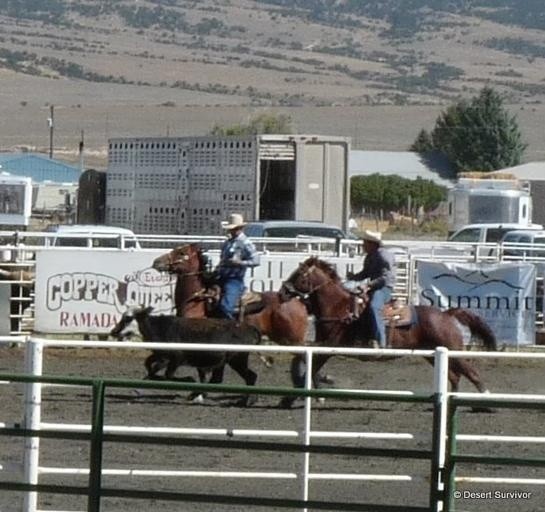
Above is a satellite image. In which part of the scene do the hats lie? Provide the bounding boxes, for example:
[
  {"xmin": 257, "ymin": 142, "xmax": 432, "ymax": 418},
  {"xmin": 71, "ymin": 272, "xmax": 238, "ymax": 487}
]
[
  {"xmin": 360, "ymin": 230, "xmax": 382, "ymax": 242},
  {"xmin": 220, "ymin": 214, "xmax": 248, "ymax": 230}
]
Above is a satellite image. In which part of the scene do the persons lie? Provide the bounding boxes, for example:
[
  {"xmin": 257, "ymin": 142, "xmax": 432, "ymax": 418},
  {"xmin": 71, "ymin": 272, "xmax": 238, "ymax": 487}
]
[
  {"xmin": 346, "ymin": 229, "xmax": 395, "ymax": 349},
  {"xmin": 210, "ymin": 213, "xmax": 261, "ymax": 319}
]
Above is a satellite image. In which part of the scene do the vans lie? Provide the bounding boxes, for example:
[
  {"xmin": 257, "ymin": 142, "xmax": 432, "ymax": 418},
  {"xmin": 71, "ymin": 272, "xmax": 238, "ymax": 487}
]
[
  {"xmin": 243, "ymin": 220, "xmax": 349, "ymax": 252},
  {"xmin": 448, "ymin": 223, "xmax": 545, "ymax": 277},
  {"xmin": 43, "ymin": 224, "xmax": 141, "ymax": 248}
]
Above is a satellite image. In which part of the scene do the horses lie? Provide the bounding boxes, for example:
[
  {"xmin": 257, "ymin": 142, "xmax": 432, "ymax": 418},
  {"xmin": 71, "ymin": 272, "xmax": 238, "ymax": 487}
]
[
  {"xmin": 152, "ymin": 240, "xmax": 336, "ymax": 406},
  {"xmin": 277, "ymin": 254, "xmax": 502, "ymax": 413}
]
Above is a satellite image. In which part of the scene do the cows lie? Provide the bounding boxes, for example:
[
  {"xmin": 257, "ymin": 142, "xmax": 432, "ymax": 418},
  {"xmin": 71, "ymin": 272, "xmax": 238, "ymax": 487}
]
[{"xmin": 110, "ymin": 304, "xmax": 262, "ymax": 406}]
